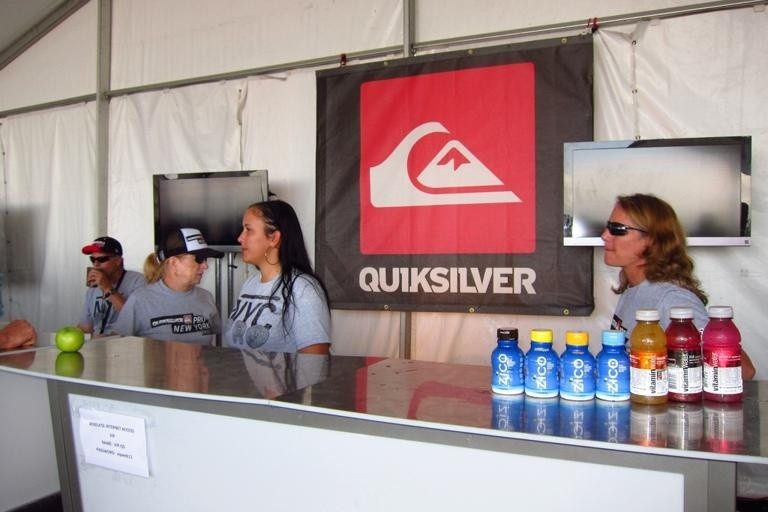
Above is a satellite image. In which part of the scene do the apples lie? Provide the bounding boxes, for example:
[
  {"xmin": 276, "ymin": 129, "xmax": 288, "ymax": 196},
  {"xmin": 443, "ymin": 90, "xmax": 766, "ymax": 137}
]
[
  {"xmin": 55, "ymin": 352, "xmax": 84, "ymax": 378},
  {"xmin": 56, "ymin": 326, "xmax": 84, "ymax": 352}
]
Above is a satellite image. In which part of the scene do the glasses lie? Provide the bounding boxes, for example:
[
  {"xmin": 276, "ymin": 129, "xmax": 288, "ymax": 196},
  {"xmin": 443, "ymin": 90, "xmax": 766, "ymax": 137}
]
[
  {"xmin": 183, "ymin": 251, "xmax": 208, "ymax": 264},
  {"xmin": 90, "ymin": 255, "xmax": 109, "ymax": 263},
  {"xmin": 606, "ymin": 220, "xmax": 649, "ymax": 236}
]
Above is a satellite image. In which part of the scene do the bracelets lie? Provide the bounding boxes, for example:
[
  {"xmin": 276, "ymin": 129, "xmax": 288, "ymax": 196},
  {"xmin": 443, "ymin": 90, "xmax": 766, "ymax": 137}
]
[{"xmin": 103, "ymin": 287, "xmax": 114, "ymax": 300}]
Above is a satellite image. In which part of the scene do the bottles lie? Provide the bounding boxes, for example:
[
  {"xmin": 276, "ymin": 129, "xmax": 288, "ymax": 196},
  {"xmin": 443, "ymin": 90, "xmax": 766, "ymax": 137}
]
[
  {"xmin": 492, "ymin": 398, "xmax": 745, "ymax": 456},
  {"xmin": 491, "ymin": 305, "xmax": 745, "ymax": 407}
]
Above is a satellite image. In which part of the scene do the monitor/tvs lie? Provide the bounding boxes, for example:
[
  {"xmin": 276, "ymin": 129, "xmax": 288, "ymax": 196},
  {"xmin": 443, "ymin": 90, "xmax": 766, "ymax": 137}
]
[
  {"xmin": 562, "ymin": 135, "xmax": 754, "ymax": 247},
  {"xmin": 151, "ymin": 169, "xmax": 269, "ymax": 251}
]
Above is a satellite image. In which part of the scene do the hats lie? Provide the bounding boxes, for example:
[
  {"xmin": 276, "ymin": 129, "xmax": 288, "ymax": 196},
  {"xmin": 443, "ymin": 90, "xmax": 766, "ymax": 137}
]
[
  {"xmin": 158, "ymin": 228, "xmax": 224, "ymax": 260},
  {"xmin": 81, "ymin": 237, "xmax": 122, "ymax": 256}
]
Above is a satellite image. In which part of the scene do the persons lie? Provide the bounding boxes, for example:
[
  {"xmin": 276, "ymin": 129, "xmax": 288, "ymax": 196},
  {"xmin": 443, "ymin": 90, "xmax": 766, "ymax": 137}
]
[
  {"xmin": 240, "ymin": 348, "xmax": 330, "ymax": 399},
  {"xmin": 0, "ymin": 319, "xmax": 38, "ymax": 351},
  {"xmin": 160, "ymin": 340, "xmax": 210, "ymax": 394},
  {"xmin": 222, "ymin": 200, "xmax": 332, "ymax": 355},
  {"xmin": 95, "ymin": 226, "xmax": 223, "ymax": 348},
  {"xmin": 76, "ymin": 236, "xmax": 147, "ymax": 336},
  {"xmin": 601, "ymin": 192, "xmax": 757, "ymax": 383}
]
